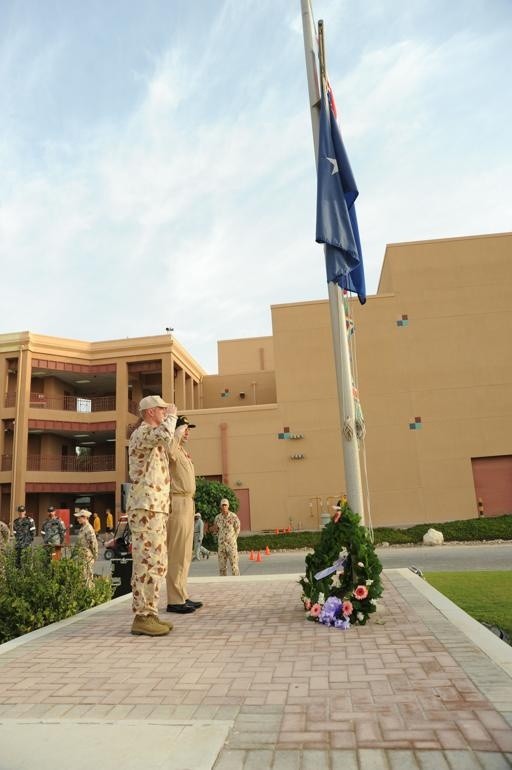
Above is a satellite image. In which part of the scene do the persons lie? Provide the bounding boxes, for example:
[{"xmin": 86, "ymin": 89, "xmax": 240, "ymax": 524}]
[
  {"xmin": 91, "ymin": 512, "xmax": 104, "ymax": 546},
  {"xmin": 192, "ymin": 512, "xmax": 211, "ymax": 563},
  {"xmin": 214, "ymin": 498, "xmax": 242, "ymax": 577},
  {"xmin": 13, "ymin": 505, "xmax": 37, "ymax": 570},
  {"xmin": 104, "ymin": 507, "xmax": 115, "ymax": 542},
  {"xmin": 40, "ymin": 505, "xmax": 67, "ymax": 565},
  {"xmin": 73, "ymin": 509, "xmax": 98, "ymax": 585},
  {"xmin": 163, "ymin": 414, "xmax": 203, "ymax": 615},
  {"xmin": 0, "ymin": 521, "xmax": 11, "ymax": 551},
  {"xmin": 127, "ymin": 394, "xmax": 175, "ymax": 636}
]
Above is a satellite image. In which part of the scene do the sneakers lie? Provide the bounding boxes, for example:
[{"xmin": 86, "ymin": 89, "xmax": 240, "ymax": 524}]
[{"xmin": 131, "ymin": 615, "xmax": 173, "ymax": 636}]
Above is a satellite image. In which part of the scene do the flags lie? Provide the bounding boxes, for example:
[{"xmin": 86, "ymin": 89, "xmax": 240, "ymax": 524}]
[{"xmin": 313, "ymin": 67, "xmax": 369, "ymax": 306}]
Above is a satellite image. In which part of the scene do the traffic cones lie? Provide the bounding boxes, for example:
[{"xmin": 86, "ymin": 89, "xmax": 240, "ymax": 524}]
[
  {"xmin": 264, "ymin": 545, "xmax": 271, "ymax": 554},
  {"xmin": 275, "ymin": 529, "xmax": 279, "ymax": 536},
  {"xmin": 249, "ymin": 550, "xmax": 255, "ymax": 560},
  {"xmin": 287, "ymin": 527, "xmax": 293, "ymax": 533},
  {"xmin": 254, "ymin": 551, "xmax": 264, "ymax": 562},
  {"xmin": 282, "ymin": 527, "xmax": 286, "ymax": 536}
]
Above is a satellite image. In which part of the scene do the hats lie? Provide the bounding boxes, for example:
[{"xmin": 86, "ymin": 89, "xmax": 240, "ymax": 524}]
[
  {"xmin": 140, "ymin": 395, "xmax": 175, "ymax": 411},
  {"xmin": 73, "ymin": 510, "xmax": 92, "ymax": 517},
  {"xmin": 176, "ymin": 415, "xmax": 195, "ymax": 428},
  {"xmin": 221, "ymin": 499, "xmax": 228, "ymax": 505}
]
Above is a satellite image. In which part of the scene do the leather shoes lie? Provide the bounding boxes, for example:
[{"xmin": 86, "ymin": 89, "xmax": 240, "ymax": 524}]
[{"xmin": 167, "ymin": 599, "xmax": 202, "ymax": 613}]
